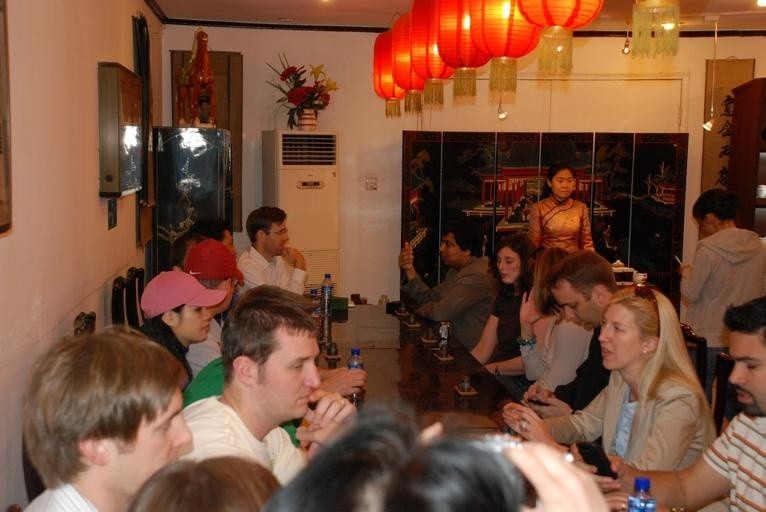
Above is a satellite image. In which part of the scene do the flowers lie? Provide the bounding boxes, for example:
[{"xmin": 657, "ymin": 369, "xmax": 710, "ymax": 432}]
[{"xmin": 266, "ymin": 51, "xmax": 341, "ymax": 132}]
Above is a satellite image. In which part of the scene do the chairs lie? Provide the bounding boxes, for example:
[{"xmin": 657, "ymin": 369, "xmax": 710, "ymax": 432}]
[
  {"xmin": 713, "ymin": 353, "xmax": 739, "ymax": 436},
  {"xmin": 111, "ymin": 265, "xmax": 146, "ymax": 332},
  {"xmin": 678, "ymin": 323, "xmax": 707, "ymax": 398}
]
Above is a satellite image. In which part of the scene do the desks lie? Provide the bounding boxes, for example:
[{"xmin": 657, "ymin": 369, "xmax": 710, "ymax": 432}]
[{"xmin": 309, "ymin": 301, "xmax": 524, "ymax": 437}]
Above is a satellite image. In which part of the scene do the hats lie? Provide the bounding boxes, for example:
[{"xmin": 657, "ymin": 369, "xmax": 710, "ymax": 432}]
[
  {"xmin": 182, "ymin": 238, "xmax": 246, "ymax": 287},
  {"xmin": 140, "ymin": 269, "xmax": 229, "ymax": 321}
]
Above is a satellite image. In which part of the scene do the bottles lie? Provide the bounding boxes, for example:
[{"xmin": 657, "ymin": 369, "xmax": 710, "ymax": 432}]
[
  {"xmin": 627, "ymin": 477, "xmax": 654, "ymax": 512},
  {"xmin": 309, "ymin": 289, "xmax": 321, "ymax": 317},
  {"xmin": 347, "ymin": 349, "xmax": 364, "ymax": 399},
  {"xmin": 321, "ymin": 274, "xmax": 333, "ymax": 317}
]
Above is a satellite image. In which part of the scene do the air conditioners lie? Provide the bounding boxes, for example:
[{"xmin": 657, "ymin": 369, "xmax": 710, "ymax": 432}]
[{"xmin": 261, "ymin": 130, "xmax": 342, "ymax": 297}]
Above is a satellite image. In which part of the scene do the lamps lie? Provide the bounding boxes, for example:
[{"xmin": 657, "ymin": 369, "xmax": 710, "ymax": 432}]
[
  {"xmin": 497, "ymin": 92, "xmax": 507, "ymax": 119},
  {"xmin": 620, "ymin": 19, "xmax": 631, "ymax": 55},
  {"xmin": 702, "ymin": 14, "xmax": 718, "ymax": 132}
]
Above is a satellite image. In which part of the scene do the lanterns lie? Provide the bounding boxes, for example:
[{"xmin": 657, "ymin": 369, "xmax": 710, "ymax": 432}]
[
  {"xmin": 437, "ymin": 1, "xmax": 482, "ymax": 106},
  {"xmin": 371, "ymin": 31, "xmax": 405, "ymax": 119},
  {"xmin": 470, "ymin": 1, "xmax": 542, "ymax": 107},
  {"xmin": 390, "ymin": 13, "xmax": 424, "ymax": 117},
  {"xmin": 518, "ymin": 1, "xmax": 605, "ymax": 80},
  {"xmin": 409, "ymin": 3, "xmax": 455, "ymax": 113}
]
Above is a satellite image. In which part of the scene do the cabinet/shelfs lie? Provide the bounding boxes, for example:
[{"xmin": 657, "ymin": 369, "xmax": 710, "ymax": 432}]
[{"xmin": 726, "ymin": 77, "xmax": 766, "ymax": 243}]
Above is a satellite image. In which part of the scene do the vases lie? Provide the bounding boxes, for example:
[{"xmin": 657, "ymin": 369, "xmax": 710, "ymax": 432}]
[{"xmin": 296, "ymin": 107, "xmax": 318, "ymax": 132}]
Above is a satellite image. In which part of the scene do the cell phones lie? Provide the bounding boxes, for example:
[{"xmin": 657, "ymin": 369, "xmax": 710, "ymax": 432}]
[
  {"xmin": 527, "ymin": 398, "xmax": 551, "ymax": 406},
  {"xmin": 576, "ymin": 441, "xmax": 618, "ymax": 481}
]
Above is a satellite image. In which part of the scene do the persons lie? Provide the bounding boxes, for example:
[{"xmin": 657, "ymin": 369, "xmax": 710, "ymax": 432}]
[
  {"xmin": 527, "ymin": 162, "xmax": 596, "ymax": 253},
  {"xmin": 0, "ymin": 187, "xmax": 766, "ymax": 512}
]
